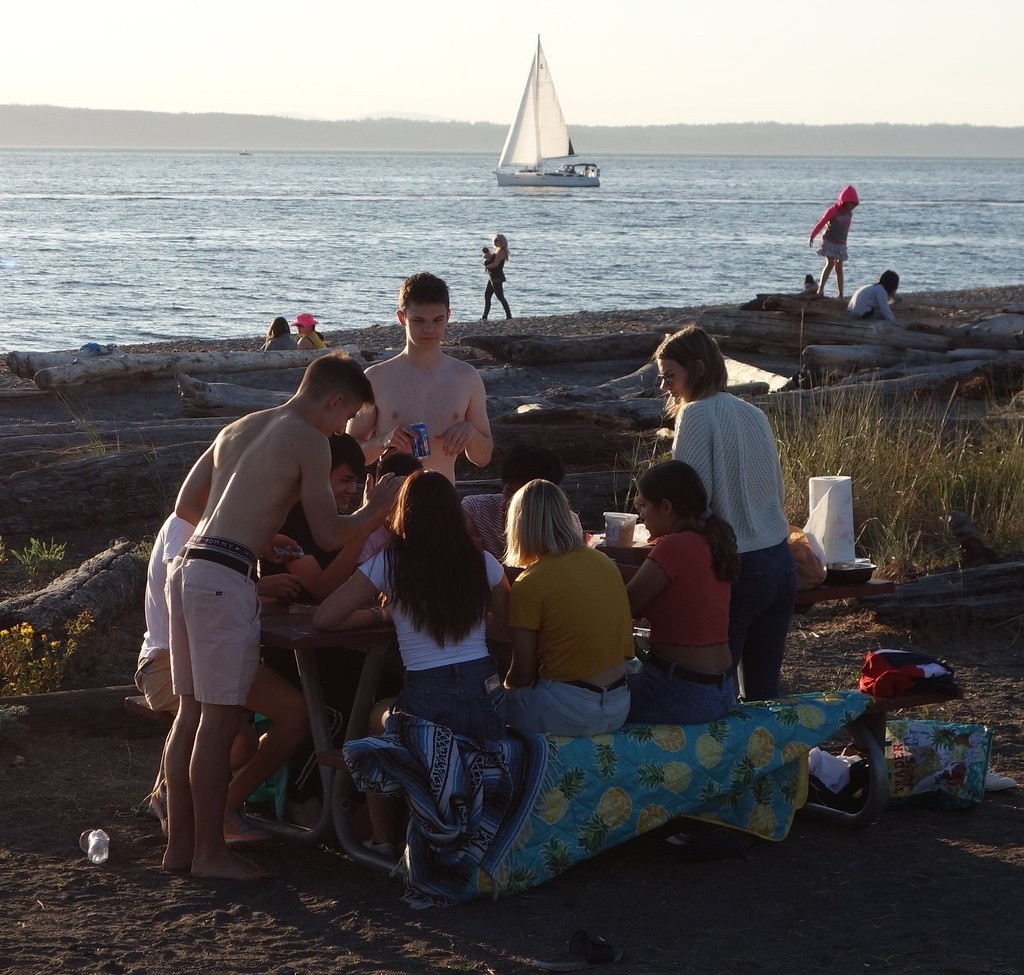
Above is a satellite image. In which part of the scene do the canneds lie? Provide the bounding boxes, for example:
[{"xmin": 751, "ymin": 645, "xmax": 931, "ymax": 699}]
[{"xmin": 408, "ymin": 422, "xmax": 431, "ymax": 459}]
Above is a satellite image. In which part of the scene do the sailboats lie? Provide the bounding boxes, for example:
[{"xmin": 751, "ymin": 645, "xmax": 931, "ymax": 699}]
[{"xmin": 490, "ymin": 33, "xmax": 601, "ymax": 188}]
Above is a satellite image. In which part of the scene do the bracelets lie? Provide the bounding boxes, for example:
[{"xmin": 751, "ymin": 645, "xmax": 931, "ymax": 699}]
[{"xmin": 369, "ymin": 606, "xmax": 388, "ymax": 625}]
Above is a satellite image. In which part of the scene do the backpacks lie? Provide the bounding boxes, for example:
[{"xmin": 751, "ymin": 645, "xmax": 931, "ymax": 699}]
[{"xmin": 858, "ymin": 649, "xmax": 956, "ymax": 698}]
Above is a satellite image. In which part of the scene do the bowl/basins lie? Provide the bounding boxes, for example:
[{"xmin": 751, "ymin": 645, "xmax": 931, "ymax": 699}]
[{"xmin": 823, "ymin": 561, "xmax": 877, "ymax": 587}]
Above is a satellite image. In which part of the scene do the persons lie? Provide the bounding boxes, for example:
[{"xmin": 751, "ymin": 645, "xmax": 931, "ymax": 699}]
[
  {"xmin": 135, "ymin": 433, "xmax": 308, "ymax": 845},
  {"xmin": 162, "ymin": 348, "xmax": 376, "ymax": 874},
  {"xmin": 290, "ymin": 312, "xmax": 328, "ymax": 350},
  {"xmin": 559, "ymin": 164, "xmax": 576, "ymax": 174},
  {"xmin": 846, "ymin": 269, "xmax": 899, "ymax": 322},
  {"xmin": 804, "ymin": 274, "xmax": 819, "ymax": 291},
  {"xmin": 344, "ymin": 271, "xmax": 494, "ymax": 487},
  {"xmin": 258, "ymin": 429, "xmax": 404, "ymax": 830},
  {"xmin": 479, "ymin": 231, "xmax": 512, "ymax": 321},
  {"xmin": 580, "ymin": 166, "xmax": 595, "ymax": 178},
  {"xmin": 482, "ymin": 246, "xmax": 492, "ymax": 273},
  {"xmin": 319, "ymin": 451, "xmax": 425, "ymax": 697},
  {"xmin": 460, "ymin": 446, "xmax": 565, "ymax": 683},
  {"xmin": 312, "ymin": 468, "xmax": 512, "ymax": 858},
  {"xmin": 809, "ymin": 185, "xmax": 859, "ymax": 298},
  {"xmin": 624, "ymin": 459, "xmax": 741, "ymax": 727},
  {"xmin": 497, "ymin": 478, "xmax": 635, "ymax": 737},
  {"xmin": 260, "ymin": 316, "xmax": 298, "ymax": 351},
  {"xmin": 650, "ymin": 319, "xmax": 796, "ymax": 703}
]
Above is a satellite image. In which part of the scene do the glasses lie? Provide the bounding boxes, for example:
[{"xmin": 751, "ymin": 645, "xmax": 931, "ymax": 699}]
[{"xmin": 658, "ymin": 368, "xmax": 685, "ymax": 386}]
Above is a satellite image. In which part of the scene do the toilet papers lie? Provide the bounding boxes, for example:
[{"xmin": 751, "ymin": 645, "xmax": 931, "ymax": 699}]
[{"xmin": 802, "ymin": 475, "xmax": 856, "ymax": 565}]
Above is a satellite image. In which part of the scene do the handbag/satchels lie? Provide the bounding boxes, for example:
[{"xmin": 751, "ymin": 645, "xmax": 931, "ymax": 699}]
[{"xmin": 886, "ymin": 719, "xmax": 992, "ymax": 810}]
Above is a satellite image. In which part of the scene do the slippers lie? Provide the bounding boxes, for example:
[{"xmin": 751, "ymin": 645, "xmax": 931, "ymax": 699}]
[{"xmin": 532, "ymin": 929, "xmax": 623, "ymax": 971}]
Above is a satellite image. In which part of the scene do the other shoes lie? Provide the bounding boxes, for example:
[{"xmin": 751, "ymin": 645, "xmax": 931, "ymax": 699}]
[
  {"xmin": 364, "ymin": 839, "xmax": 396, "ymax": 859},
  {"xmin": 987, "ymin": 773, "xmax": 1018, "ymax": 792}
]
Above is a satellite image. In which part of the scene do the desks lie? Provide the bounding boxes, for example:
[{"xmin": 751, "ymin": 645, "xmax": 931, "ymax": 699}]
[{"xmin": 209, "ymin": 574, "xmax": 889, "ymax": 887}]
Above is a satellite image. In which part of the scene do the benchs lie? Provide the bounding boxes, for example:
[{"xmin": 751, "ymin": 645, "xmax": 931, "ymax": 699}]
[
  {"xmin": 121, "ymin": 692, "xmax": 272, "ymax": 736},
  {"xmin": 342, "ymin": 683, "xmax": 961, "ymax": 904}
]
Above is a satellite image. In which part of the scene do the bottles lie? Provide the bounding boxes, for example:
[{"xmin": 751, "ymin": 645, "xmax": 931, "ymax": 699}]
[{"xmin": 88, "ymin": 828, "xmax": 110, "ymax": 864}]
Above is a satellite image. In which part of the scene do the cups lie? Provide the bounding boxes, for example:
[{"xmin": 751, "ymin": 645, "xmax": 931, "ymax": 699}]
[{"xmin": 603, "ymin": 511, "xmax": 640, "ymax": 548}]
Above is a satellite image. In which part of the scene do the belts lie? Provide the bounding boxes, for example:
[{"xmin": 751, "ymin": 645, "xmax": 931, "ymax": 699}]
[
  {"xmin": 181, "ymin": 549, "xmax": 258, "ymax": 583},
  {"xmin": 648, "ymin": 653, "xmax": 733, "ymax": 685},
  {"xmin": 566, "ymin": 675, "xmax": 626, "ymax": 693}
]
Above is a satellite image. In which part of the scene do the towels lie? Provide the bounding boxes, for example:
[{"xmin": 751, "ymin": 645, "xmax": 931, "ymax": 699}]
[{"xmin": 808, "ymin": 745, "xmax": 862, "ymax": 794}]
